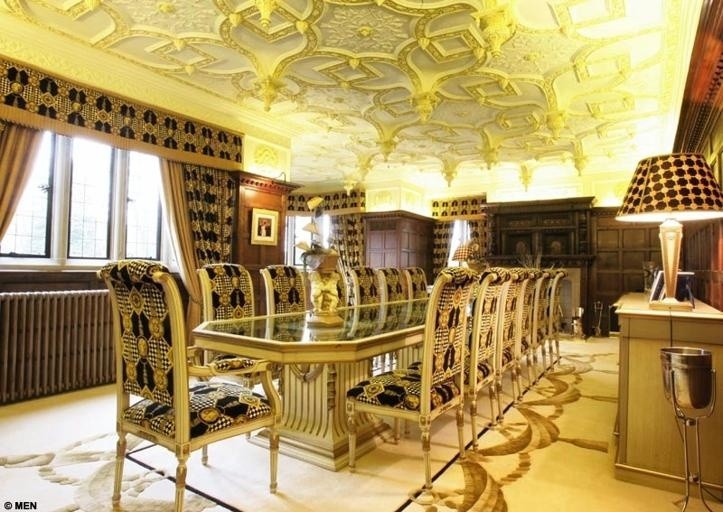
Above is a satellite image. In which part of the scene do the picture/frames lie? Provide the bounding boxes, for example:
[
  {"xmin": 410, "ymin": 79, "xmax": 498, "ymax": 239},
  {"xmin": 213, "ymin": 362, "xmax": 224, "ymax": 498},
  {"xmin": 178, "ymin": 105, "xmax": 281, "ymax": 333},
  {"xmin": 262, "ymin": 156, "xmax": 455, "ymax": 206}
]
[{"xmin": 249, "ymin": 207, "xmax": 279, "ymax": 247}]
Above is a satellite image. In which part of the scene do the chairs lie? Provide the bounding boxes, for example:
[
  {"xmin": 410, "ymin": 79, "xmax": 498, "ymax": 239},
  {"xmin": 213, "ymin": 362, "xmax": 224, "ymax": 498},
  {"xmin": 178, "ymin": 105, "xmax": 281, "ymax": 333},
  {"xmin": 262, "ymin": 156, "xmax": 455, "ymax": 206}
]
[{"xmin": 95, "ymin": 259, "xmax": 567, "ymax": 512}]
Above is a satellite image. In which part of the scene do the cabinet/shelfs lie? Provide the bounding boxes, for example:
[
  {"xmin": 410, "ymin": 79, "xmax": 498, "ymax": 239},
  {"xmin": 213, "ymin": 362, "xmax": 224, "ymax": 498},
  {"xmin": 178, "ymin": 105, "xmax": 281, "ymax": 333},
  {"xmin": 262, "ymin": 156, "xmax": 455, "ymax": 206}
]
[{"xmin": 614, "ymin": 292, "xmax": 722, "ymax": 503}]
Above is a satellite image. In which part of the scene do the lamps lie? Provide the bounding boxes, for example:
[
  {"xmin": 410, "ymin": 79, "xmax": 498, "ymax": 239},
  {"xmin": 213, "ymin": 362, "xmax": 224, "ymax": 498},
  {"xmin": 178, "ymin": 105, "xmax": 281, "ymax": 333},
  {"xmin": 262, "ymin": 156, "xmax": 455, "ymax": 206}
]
[{"xmin": 612, "ymin": 152, "xmax": 723, "ymax": 311}]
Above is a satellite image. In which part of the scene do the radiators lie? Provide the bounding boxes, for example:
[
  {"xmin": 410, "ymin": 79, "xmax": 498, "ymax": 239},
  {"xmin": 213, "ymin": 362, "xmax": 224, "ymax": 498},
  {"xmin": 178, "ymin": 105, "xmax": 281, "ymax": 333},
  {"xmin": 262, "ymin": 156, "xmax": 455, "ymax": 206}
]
[{"xmin": 0, "ymin": 289, "xmax": 116, "ymax": 403}]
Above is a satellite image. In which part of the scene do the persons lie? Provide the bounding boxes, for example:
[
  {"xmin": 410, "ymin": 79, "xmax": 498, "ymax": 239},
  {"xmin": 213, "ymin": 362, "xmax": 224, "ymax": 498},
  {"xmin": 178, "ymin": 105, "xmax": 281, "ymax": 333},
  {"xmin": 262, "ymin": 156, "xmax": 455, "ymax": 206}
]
[
  {"xmin": 324, "ymin": 272, "xmax": 341, "ymax": 312},
  {"xmin": 308, "ymin": 271, "xmax": 323, "ymax": 312}
]
